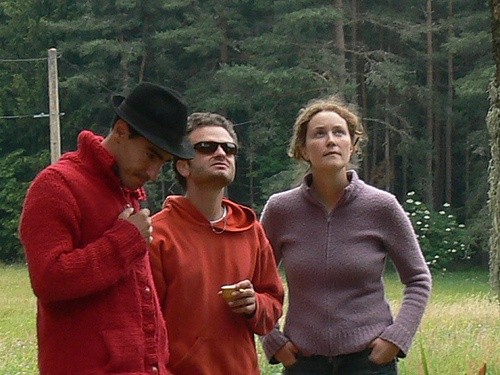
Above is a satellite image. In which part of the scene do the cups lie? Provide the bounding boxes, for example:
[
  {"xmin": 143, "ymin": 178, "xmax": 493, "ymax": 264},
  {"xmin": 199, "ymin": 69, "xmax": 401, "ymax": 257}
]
[{"xmin": 221, "ymin": 287, "xmax": 242, "ymax": 301}]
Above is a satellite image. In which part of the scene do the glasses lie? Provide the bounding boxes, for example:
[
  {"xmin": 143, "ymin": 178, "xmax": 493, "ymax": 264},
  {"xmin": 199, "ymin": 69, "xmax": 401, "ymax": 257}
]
[{"xmin": 193, "ymin": 141, "xmax": 238, "ymax": 156}]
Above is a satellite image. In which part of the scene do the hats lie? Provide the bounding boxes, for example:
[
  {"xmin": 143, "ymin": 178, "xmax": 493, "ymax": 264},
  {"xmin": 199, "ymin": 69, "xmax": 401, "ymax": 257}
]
[{"xmin": 110, "ymin": 82, "xmax": 193, "ymax": 161}]
